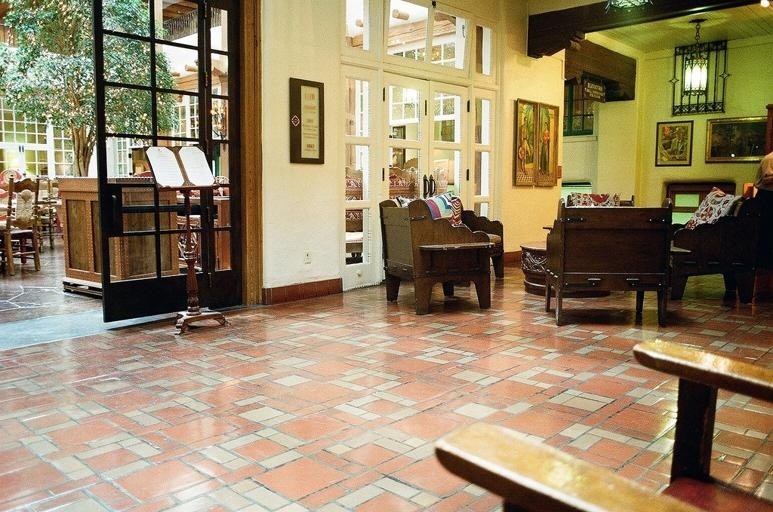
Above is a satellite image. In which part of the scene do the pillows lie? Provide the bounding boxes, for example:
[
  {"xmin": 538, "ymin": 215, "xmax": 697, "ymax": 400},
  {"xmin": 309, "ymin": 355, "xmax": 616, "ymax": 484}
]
[
  {"xmin": 408, "ymin": 192, "xmax": 460, "ymax": 228},
  {"xmin": 686, "ymin": 188, "xmax": 738, "ymax": 232},
  {"xmin": 570, "ymin": 192, "xmax": 620, "ymax": 206}
]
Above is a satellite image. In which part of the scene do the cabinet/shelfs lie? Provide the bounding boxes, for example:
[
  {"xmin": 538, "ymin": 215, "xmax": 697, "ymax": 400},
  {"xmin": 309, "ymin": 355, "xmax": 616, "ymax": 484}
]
[{"xmin": 57, "ymin": 176, "xmax": 179, "ymax": 283}]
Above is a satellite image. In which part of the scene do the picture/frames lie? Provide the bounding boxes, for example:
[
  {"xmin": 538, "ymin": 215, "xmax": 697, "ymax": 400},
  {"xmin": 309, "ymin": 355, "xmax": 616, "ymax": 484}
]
[
  {"xmin": 656, "ymin": 120, "xmax": 693, "ymax": 167},
  {"xmin": 289, "ymin": 78, "xmax": 325, "ymax": 166},
  {"xmin": 513, "ymin": 97, "xmax": 536, "ymax": 187},
  {"xmin": 705, "ymin": 117, "xmax": 767, "ymax": 161},
  {"xmin": 536, "ymin": 103, "xmax": 559, "ymax": 187}
]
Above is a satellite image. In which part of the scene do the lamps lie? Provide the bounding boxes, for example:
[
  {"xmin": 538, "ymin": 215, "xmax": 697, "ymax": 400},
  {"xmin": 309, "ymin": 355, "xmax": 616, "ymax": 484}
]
[{"xmin": 672, "ymin": 20, "xmax": 730, "ymax": 114}]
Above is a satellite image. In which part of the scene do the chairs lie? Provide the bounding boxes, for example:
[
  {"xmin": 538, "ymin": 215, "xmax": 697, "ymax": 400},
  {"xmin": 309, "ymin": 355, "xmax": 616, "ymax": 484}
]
[
  {"xmin": 434, "ymin": 340, "xmax": 773, "ymax": 511},
  {"xmin": 674, "ymin": 189, "xmax": 760, "ymax": 306},
  {"xmin": 400, "ymin": 195, "xmax": 508, "ymax": 277},
  {"xmin": 0, "ymin": 177, "xmax": 41, "ymax": 276},
  {"xmin": 544, "ymin": 199, "xmax": 673, "ymax": 326},
  {"xmin": 379, "ymin": 201, "xmax": 496, "ymax": 314}
]
